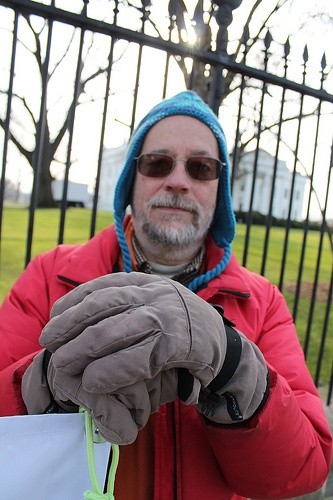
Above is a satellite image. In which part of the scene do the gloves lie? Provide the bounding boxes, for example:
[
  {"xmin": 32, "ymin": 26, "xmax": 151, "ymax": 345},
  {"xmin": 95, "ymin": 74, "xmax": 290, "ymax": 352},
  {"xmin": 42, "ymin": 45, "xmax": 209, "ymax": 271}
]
[
  {"xmin": 37, "ymin": 270, "xmax": 270, "ymax": 427},
  {"xmin": 21, "ymin": 344, "xmax": 203, "ymax": 447}
]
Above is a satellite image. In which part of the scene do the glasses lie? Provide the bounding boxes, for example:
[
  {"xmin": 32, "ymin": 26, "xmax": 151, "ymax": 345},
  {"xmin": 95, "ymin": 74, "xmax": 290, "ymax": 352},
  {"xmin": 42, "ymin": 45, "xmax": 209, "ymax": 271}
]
[{"xmin": 133, "ymin": 152, "xmax": 228, "ymax": 181}]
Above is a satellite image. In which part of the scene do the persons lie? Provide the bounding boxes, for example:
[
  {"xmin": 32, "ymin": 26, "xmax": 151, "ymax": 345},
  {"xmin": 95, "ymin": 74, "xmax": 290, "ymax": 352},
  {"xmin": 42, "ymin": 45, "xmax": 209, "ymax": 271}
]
[{"xmin": 0, "ymin": 88, "xmax": 333, "ymax": 500}]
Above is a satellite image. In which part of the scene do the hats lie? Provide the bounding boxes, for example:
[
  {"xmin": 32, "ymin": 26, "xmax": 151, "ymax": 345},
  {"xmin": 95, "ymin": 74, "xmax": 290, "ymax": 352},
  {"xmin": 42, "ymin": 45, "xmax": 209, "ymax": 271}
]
[{"xmin": 113, "ymin": 89, "xmax": 237, "ymax": 292}]
